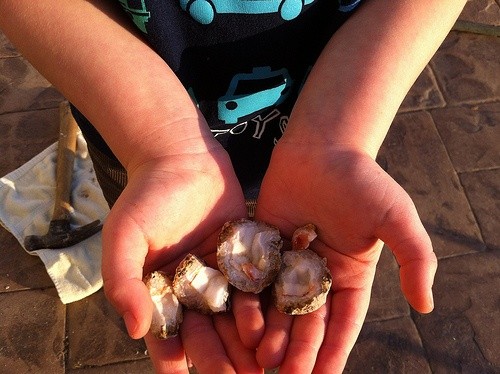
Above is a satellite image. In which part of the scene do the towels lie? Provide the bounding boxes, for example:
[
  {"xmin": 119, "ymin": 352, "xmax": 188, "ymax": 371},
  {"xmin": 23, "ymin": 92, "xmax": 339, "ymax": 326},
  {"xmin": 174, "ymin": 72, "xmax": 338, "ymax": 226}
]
[{"xmin": 0, "ymin": 130, "xmax": 111, "ymax": 304}]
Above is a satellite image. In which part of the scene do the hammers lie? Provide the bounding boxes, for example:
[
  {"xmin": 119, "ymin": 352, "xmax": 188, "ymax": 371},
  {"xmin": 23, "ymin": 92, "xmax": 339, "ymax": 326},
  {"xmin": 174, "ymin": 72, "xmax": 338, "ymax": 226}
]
[{"xmin": 24, "ymin": 100, "xmax": 104, "ymax": 252}]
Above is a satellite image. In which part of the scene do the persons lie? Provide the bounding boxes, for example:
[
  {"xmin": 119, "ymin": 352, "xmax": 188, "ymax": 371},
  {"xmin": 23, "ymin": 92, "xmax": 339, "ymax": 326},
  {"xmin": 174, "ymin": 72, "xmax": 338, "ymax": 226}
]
[{"xmin": 0, "ymin": 1, "xmax": 467, "ymax": 374}]
